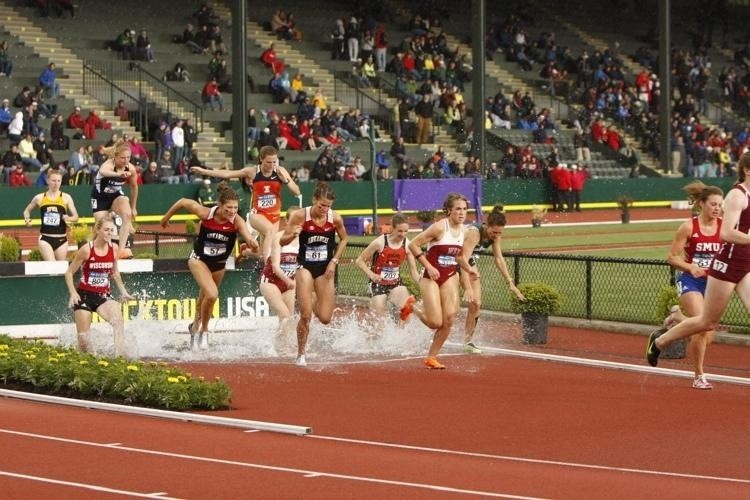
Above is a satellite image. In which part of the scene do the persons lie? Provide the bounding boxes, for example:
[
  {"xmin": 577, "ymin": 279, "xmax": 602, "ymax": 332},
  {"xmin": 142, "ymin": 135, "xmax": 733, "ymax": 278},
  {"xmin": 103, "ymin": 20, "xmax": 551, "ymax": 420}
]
[
  {"xmin": 190, "ymin": 146, "xmax": 300, "ymax": 266},
  {"xmin": 280, "ymin": 183, "xmax": 348, "ymax": 367},
  {"xmin": 91, "ymin": 141, "xmax": 138, "ymax": 259},
  {"xmin": 647, "ymin": 152, "xmax": 750, "ymax": 390},
  {"xmin": 356, "ymin": 214, "xmax": 420, "ymax": 348},
  {"xmin": 65, "ymin": 216, "xmax": 135, "ymax": 357},
  {"xmin": 161, "ymin": 179, "xmax": 259, "ymax": 351},
  {"xmin": 23, "ymin": 168, "xmax": 80, "ymax": 261},
  {"xmin": 668, "ymin": 179, "xmax": 727, "ymax": 390},
  {"xmin": 259, "ymin": 207, "xmax": 303, "ymax": 360},
  {"xmin": 398, "ymin": 192, "xmax": 479, "ymax": 369},
  {"xmin": 453, "ymin": 207, "xmax": 526, "ymax": 355}
]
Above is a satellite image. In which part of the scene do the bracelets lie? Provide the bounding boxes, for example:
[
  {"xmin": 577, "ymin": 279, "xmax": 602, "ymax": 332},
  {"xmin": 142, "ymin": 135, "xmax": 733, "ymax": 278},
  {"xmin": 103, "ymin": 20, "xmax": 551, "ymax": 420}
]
[
  {"xmin": 505, "ymin": 278, "xmax": 514, "ymax": 285},
  {"xmin": 415, "ymin": 253, "xmax": 427, "ymax": 259}
]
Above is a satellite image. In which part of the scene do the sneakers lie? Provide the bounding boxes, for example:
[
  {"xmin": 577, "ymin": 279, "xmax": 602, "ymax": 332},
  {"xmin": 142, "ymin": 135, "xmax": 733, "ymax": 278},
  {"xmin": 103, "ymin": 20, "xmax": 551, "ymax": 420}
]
[
  {"xmin": 693, "ymin": 374, "xmax": 713, "ymax": 390},
  {"xmin": 400, "ymin": 295, "xmax": 416, "ymax": 320},
  {"xmin": 198, "ymin": 330, "xmax": 210, "ymax": 348},
  {"xmin": 662, "ymin": 305, "xmax": 681, "ymax": 329},
  {"xmin": 295, "ymin": 354, "xmax": 308, "ymax": 367},
  {"xmin": 188, "ymin": 323, "xmax": 200, "ymax": 351},
  {"xmin": 464, "ymin": 342, "xmax": 482, "ymax": 354},
  {"xmin": 425, "ymin": 357, "xmax": 446, "ymax": 370},
  {"xmin": 647, "ymin": 330, "xmax": 661, "ymax": 368}
]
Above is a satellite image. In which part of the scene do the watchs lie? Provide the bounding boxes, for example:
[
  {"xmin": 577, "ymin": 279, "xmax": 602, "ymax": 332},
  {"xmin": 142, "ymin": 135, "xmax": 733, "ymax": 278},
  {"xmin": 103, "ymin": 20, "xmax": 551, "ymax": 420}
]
[{"xmin": 332, "ymin": 258, "xmax": 339, "ymax": 265}]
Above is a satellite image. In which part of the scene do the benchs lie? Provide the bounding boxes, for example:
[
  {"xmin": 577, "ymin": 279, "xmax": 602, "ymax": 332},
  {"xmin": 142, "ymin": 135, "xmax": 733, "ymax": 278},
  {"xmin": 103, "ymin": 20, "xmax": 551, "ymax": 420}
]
[
  {"xmin": 486, "ymin": 2, "xmax": 662, "ymax": 175},
  {"xmin": 670, "ymin": 10, "xmax": 750, "ymax": 133},
  {"xmin": 246, "ymin": 2, "xmax": 474, "ymax": 169},
  {"xmin": 2, "ymin": 3, "xmax": 235, "ymax": 170}
]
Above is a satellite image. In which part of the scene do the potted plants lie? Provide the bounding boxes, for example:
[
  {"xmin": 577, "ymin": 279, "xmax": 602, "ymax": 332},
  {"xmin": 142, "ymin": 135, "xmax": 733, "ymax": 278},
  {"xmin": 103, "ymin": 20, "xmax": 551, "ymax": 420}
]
[
  {"xmin": 530, "ymin": 205, "xmax": 548, "ymax": 228},
  {"xmin": 69, "ymin": 222, "xmax": 91, "ymax": 250},
  {"xmin": 651, "ymin": 286, "xmax": 690, "ymax": 360},
  {"xmin": 183, "ymin": 220, "xmax": 199, "ymax": 243},
  {"xmin": 509, "ymin": 281, "xmax": 564, "ymax": 349},
  {"xmin": 416, "ymin": 208, "xmax": 437, "ymax": 232},
  {"xmin": 615, "ymin": 196, "xmax": 632, "ymax": 225}
]
